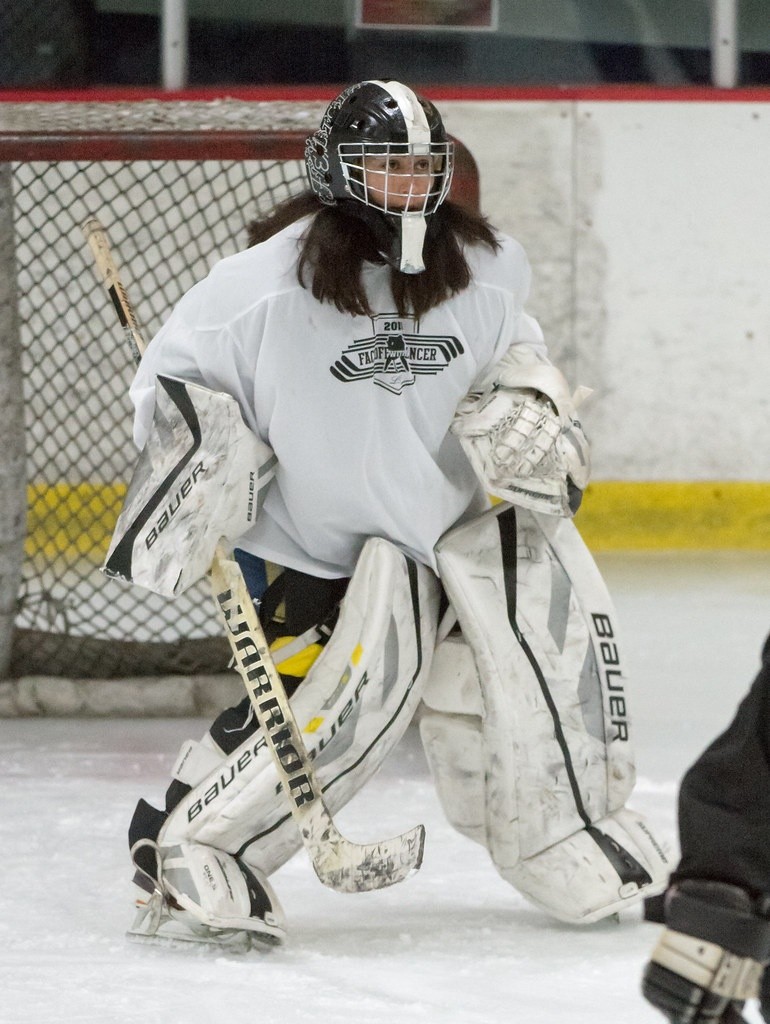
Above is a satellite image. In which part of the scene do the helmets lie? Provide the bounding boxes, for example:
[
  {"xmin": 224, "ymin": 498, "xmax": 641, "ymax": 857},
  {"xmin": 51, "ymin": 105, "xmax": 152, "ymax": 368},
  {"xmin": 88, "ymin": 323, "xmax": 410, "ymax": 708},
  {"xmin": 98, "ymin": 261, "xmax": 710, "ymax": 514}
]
[{"xmin": 303, "ymin": 80, "xmax": 455, "ymax": 218}]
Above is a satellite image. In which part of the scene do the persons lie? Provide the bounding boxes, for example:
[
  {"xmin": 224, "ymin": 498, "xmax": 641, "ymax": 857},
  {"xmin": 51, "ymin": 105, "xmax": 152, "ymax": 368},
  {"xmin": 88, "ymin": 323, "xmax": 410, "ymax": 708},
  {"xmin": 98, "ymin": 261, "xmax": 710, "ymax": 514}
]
[
  {"xmin": 109, "ymin": 73, "xmax": 671, "ymax": 937},
  {"xmin": 643, "ymin": 632, "xmax": 770, "ymax": 1024}
]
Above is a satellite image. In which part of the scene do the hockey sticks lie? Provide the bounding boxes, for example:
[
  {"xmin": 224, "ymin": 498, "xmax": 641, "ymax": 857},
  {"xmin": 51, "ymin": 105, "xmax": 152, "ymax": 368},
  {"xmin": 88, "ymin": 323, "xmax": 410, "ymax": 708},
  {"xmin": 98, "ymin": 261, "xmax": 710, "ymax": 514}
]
[{"xmin": 82, "ymin": 213, "xmax": 423, "ymax": 892}]
